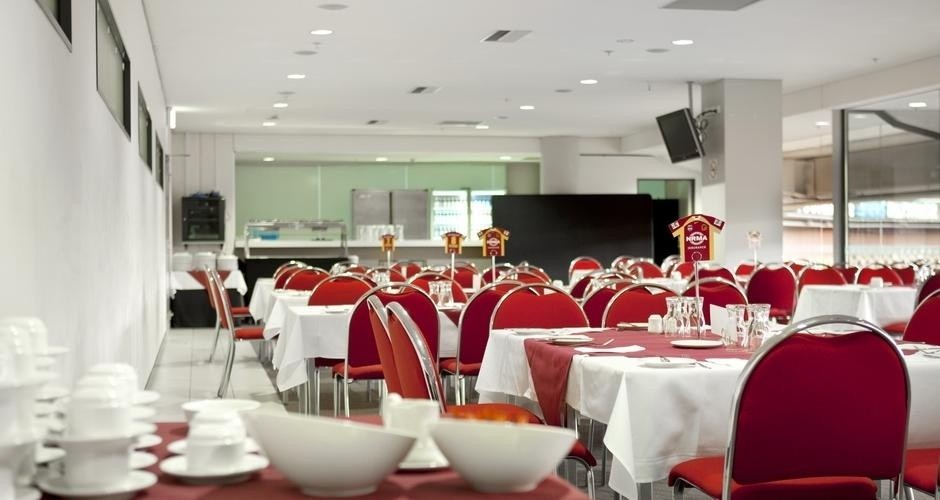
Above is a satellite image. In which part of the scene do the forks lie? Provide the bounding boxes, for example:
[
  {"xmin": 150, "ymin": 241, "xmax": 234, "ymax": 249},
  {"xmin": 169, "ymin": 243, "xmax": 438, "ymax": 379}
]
[
  {"xmin": 546, "ymin": 338, "xmax": 615, "ymax": 347},
  {"xmin": 914, "ymin": 344, "xmax": 940, "ymax": 354}
]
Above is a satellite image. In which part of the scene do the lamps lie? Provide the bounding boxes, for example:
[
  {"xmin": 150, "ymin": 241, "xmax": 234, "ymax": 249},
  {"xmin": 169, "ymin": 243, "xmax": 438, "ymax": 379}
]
[{"xmin": 168, "ymin": 108, "xmax": 178, "ymax": 130}]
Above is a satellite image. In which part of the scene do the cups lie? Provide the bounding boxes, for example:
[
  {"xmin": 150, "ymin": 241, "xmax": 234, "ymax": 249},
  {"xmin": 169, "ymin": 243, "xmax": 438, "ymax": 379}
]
[
  {"xmin": 188, "ymin": 409, "xmax": 247, "ymax": 438},
  {"xmin": 723, "ymin": 301, "xmax": 772, "ymax": 354},
  {"xmin": 185, "ymin": 426, "xmax": 242, "ymax": 473},
  {"xmin": 870, "ymin": 277, "xmax": 883, "ymax": 288},
  {"xmin": 380, "ymin": 397, "xmax": 438, "ymax": 460},
  {"xmin": 0, "ymin": 320, "xmax": 39, "ymax": 498},
  {"xmin": 374, "ymin": 272, "xmax": 391, "ymax": 293},
  {"xmin": 665, "ymin": 295, "xmax": 707, "ymax": 337},
  {"xmin": 647, "ymin": 314, "xmax": 663, "ymax": 334},
  {"xmin": 428, "ymin": 280, "xmax": 455, "ymax": 307},
  {"xmin": 60, "ymin": 362, "xmax": 141, "ymax": 484}
]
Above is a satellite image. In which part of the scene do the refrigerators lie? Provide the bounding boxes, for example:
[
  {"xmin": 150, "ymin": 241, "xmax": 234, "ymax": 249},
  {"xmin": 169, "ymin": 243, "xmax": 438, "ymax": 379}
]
[
  {"xmin": 428, "ymin": 187, "xmax": 470, "ymax": 241},
  {"xmin": 470, "ymin": 188, "xmax": 507, "ymax": 241}
]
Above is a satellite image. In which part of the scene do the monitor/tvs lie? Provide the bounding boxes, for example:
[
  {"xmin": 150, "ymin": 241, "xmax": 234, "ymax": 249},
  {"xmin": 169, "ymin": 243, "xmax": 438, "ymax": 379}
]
[{"xmin": 656, "ymin": 108, "xmax": 705, "ymax": 164}]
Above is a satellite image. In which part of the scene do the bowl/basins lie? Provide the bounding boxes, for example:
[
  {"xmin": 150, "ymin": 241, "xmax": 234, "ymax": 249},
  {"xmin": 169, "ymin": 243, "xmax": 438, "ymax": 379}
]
[
  {"xmin": 425, "ymin": 419, "xmax": 582, "ymax": 495},
  {"xmin": 237, "ymin": 407, "xmax": 417, "ymax": 496}
]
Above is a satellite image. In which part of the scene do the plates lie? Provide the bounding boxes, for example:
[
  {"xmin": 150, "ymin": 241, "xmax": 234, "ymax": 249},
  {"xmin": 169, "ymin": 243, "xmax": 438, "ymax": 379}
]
[
  {"xmin": 545, "ymin": 334, "xmax": 592, "ymax": 345},
  {"xmin": 398, "ymin": 446, "xmax": 452, "ymax": 470},
  {"xmin": 38, "ymin": 382, "xmax": 161, "ymax": 499},
  {"xmin": 180, "ymin": 398, "xmax": 262, "ymax": 420},
  {"xmin": 172, "ymin": 252, "xmax": 239, "ymax": 271},
  {"xmin": 641, "ymin": 355, "xmax": 696, "ymax": 370},
  {"xmin": 0, "ymin": 344, "xmax": 66, "ymax": 500},
  {"xmin": 157, "ymin": 452, "xmax": 267, "ymax": 482},
  {"xmin": 617, "ymin": 323, "xmax": 648, "ymax": 330},
  {"xmin": 670, "ymin": 337, "xmax": 724, "ymax": 350},
  {"xmin": 166, "ymin": 434, "xmax": 260, "ymax": 455},
  {"xmin": 324, "ymin": 306, "xmax": 350, "ymax": 314}
]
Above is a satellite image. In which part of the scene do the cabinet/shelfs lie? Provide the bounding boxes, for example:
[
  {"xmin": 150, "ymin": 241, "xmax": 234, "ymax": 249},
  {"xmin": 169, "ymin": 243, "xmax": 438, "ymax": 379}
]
[{"xmin": 351, "ymin": 187, "xmax": 430, "ymax": 240}]
